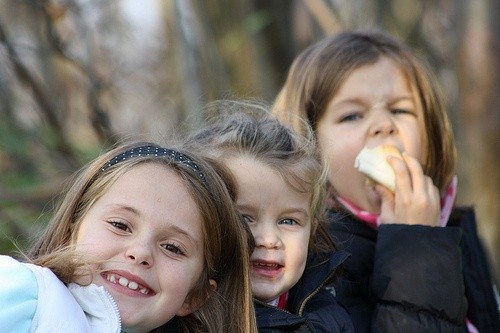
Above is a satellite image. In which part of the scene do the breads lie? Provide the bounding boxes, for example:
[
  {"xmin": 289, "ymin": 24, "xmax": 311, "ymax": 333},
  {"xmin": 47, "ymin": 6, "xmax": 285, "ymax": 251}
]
[{"xmin": 353, "ymin": 145, "xmax": 406, "ymax": 195}]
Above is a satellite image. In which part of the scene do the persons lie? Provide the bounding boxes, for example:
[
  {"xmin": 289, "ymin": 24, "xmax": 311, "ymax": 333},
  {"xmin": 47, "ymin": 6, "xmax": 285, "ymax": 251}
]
[
  {"xmin": 274, "ymin": 26, "xmax": 500, "ymax": 333},
  {"xmin": 0, "ymin": 129, "xmax": 258, "ymax": 333},
  {"xmin": 167, "ymin": 96, "xmax": 356, "ymax": 333}
]
